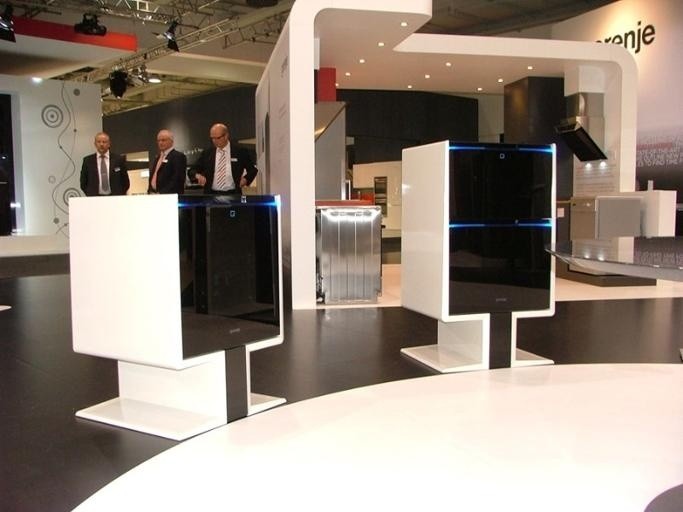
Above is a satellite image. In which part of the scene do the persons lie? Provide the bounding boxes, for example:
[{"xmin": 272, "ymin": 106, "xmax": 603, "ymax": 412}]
[
  {"xmin": 188, "ymin": 124, "xmax": 258, "ymax": 195},
  {"xmin": 80, "ymin": 131, "xmax": 130, "ymax": 197},
  {"xmin": 146, "ymin": 130, "xmax": 187, "ymax": 195}
]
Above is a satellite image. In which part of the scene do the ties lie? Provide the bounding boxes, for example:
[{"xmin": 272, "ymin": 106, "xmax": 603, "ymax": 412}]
[
  {"xmin": 215, "ymin": 148, "xmax": 227, "ymax": 191},
  {"xmin": 99, "ymin": 154, "xmax": 109, "ymax": 192},
  {"xmin": 149, "ymin": 150, "xmax": 165, "ymax": 192}
]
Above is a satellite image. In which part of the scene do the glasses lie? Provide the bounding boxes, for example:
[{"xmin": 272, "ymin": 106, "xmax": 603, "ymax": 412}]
[{"xmin": 209, "ymin": 134, "xmax": 226, "ymax": 139}]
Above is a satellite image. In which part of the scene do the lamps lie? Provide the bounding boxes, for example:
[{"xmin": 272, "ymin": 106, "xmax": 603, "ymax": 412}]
[
  {"xmin": 73, "ymin": 13, "xmax": 106, "ymax": 36},
  {"xmin": 107, "ymin": 71, "xmax": 128, "ymax": 99},
  {"xmin": 0, "ymin": 5, "xmax": 15, "ymax": 43},
  {"xmin": 164, "ymin": 20, "xmax": 178, "ymax": 53}
]
